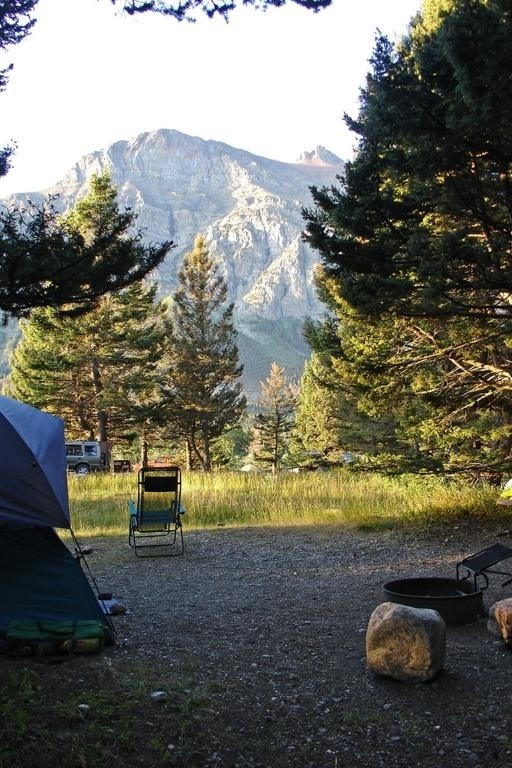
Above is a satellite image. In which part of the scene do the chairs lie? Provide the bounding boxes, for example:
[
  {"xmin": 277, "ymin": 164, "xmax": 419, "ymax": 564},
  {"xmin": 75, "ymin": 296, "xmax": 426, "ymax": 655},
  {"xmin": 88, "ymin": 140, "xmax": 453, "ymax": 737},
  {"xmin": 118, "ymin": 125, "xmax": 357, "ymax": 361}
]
[{"xmin": 128, "ymin": 467, "xmax": 186, "ymax": 558}]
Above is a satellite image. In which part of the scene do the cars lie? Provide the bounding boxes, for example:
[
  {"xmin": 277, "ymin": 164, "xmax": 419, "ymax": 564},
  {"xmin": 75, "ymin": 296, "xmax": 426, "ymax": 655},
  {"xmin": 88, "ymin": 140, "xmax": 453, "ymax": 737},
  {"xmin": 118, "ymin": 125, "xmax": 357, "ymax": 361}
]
[{"xmin": 64, "ymin": 440, "xmax": 111, "ymax": 475}]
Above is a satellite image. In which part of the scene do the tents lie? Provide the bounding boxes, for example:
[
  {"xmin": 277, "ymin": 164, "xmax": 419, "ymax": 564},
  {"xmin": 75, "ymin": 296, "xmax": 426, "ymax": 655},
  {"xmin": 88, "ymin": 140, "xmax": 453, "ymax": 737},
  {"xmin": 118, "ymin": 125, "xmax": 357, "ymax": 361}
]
[{"xmin": 1, "ymin": 393, "xmax": 119, "ymax": 655}]
